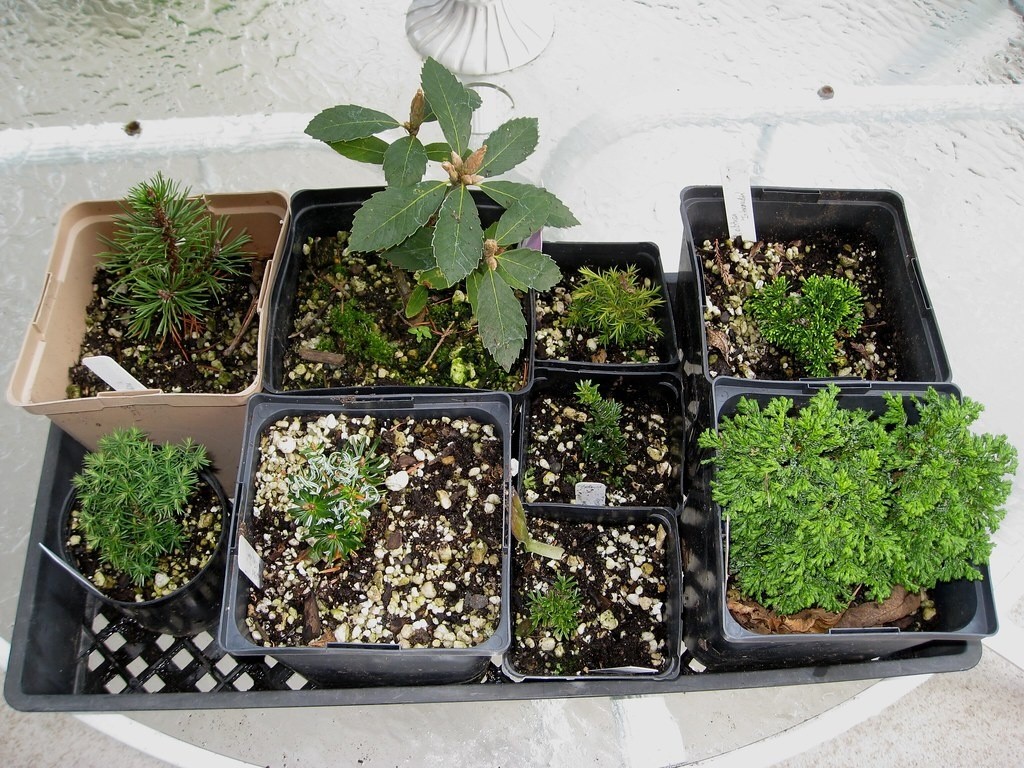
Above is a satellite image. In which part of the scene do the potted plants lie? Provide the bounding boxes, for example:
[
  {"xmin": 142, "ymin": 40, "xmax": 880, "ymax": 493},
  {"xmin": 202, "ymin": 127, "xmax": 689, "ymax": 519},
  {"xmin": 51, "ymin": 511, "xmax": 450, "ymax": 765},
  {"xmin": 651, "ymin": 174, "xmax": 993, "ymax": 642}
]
[{"xmin": 3, "ymin": 56, "xmax": 1019, "ymax": 682}]
